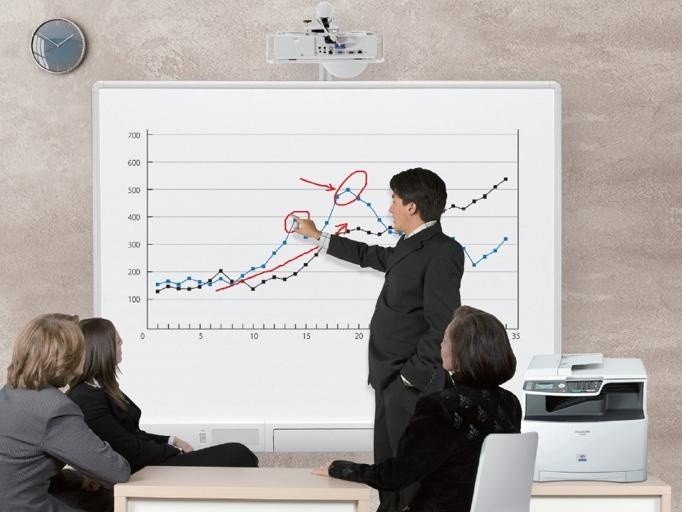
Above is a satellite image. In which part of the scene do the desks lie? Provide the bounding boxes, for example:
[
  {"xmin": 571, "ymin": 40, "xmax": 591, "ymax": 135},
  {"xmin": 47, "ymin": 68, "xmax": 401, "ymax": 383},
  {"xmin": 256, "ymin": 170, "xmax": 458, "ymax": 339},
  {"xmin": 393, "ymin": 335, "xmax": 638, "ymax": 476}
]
[{"xmin": 112, "ymin": 465, "xmax": 380, "ymax": 512}]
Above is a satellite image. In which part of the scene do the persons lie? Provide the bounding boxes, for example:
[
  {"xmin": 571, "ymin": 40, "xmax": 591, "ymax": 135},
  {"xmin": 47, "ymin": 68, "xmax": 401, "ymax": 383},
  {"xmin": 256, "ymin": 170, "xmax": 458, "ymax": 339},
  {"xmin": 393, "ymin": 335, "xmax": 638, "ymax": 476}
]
[
  {"xmin": 289, "ymin": 166, "xmax": 466, "ymax": 510},
  {"xmin": 312, "ymin": 305, "xmax": 523, "ymax": 512},
  {"xmin": 63, "ymin": 317, "xmax": 261, "ymax": 475},
  {"xmin": 1, "ymin": 312, "xmax": 133, "ymax": 511}
]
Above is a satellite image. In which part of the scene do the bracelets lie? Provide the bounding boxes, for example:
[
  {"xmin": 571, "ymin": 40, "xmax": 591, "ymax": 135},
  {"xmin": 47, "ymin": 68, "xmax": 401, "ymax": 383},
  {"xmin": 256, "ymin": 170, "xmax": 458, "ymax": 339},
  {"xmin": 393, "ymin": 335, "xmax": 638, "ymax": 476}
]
[{"xmin": 174, "ymin": 444, "xmax": 185, "ymax": 457}]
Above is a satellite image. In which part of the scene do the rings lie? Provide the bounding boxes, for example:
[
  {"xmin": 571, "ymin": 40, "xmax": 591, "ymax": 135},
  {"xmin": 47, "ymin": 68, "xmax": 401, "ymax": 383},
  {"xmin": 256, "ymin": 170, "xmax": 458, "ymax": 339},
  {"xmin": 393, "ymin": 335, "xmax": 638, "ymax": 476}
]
[{"xmin": 320, "ymin": 465, "xmax": 323, "ymax": 469}]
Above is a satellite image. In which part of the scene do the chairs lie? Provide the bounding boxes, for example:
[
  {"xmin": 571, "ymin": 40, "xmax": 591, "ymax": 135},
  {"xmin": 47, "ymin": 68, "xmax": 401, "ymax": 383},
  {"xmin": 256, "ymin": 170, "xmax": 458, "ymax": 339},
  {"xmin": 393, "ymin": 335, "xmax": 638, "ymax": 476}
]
[{"xmin": 466, "ymin": 432, "xmax": 540, "ymax": 512}]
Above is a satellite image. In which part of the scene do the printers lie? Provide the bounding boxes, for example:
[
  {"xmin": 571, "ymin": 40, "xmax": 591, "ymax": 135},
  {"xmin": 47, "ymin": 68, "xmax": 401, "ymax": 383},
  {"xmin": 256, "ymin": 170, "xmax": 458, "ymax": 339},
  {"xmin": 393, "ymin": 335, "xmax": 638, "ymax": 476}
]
[{"xmin": 520, "ymin": 351, "xmax": 650, "ymax": 483}]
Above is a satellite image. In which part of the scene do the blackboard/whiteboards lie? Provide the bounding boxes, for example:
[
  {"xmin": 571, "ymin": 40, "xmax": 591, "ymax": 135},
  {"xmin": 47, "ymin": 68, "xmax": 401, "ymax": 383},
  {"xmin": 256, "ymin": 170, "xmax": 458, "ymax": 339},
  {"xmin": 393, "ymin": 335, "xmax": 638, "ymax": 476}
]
[{"xmin": 92, "ymin": 81, "xmax": 563, "ymax": 431}]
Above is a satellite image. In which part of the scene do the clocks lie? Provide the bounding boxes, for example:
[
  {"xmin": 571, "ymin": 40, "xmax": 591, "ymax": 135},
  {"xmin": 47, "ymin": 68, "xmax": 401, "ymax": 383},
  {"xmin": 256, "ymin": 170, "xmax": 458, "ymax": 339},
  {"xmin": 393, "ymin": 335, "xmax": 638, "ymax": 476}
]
[{"xmin": 30, "ymin": 17, "xmax": 87, "ymax": 72}]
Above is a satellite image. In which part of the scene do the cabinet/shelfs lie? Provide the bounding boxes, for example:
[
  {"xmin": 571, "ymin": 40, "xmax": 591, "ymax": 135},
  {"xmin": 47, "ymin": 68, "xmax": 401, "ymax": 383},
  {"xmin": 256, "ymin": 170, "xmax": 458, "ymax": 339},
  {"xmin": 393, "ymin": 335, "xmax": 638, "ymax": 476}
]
[{"xmin": 525, "ymin": 471, "xmax": 671, "ymax": 511}]
